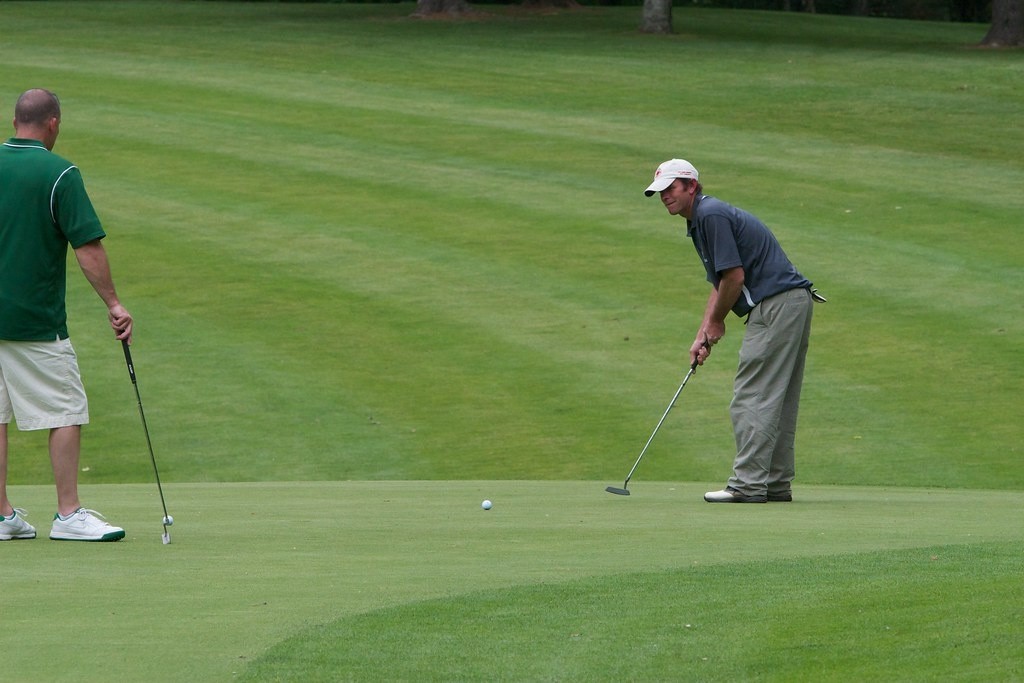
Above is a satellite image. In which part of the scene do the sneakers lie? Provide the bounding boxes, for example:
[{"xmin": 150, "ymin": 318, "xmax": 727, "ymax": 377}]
[
  {"xmin": 0, "ymin": 508, "xmax": 36, "ymax": 540},
  {"xmin": 49, "ymin": 507, "xmax": 125, "ymax": 541}
]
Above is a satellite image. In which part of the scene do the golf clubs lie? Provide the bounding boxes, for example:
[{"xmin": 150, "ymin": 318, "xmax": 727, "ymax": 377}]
[
  {"xmin": 116, "ymin": 328, "xmax": 170, "ymax": 545},
  {"xmin": 604, "ymin": 342, "xmax": 707, "ymax": 496}
]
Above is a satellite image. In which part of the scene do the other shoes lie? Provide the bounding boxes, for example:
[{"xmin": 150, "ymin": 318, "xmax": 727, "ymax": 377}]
[{"xmin": 704, "ymin": 485, "xmax": 792, "ymax": 502}]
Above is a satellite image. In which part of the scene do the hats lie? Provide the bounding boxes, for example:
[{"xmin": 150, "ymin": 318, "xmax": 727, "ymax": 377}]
[{"xmin": 644, "ymin": 158, "xmax": 698, "ymax": 197}]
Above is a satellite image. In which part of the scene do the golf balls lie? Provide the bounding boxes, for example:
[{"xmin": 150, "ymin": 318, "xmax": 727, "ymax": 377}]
[
  {"xmin": 163, "ymin": 515, "xmax": 173, "ymax": 526},
  {"xmin": 482, "ymin": 499, "xmax": 492, "ymax": 510}
]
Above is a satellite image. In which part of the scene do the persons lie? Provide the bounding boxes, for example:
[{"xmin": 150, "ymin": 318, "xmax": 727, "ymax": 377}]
[
  {"xmin": 0, "ymin": 88, "xmax": 132, "ymax": 541},
  {"xmin": 644, "ymin": 159, "xmax": 827, "ymax": 502}
]
